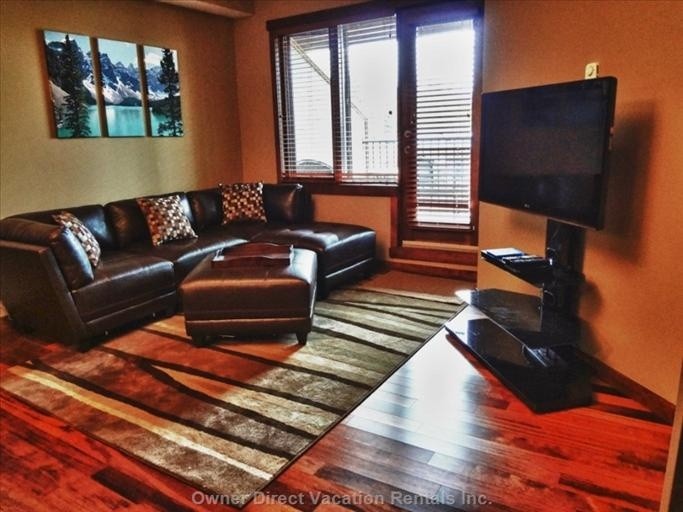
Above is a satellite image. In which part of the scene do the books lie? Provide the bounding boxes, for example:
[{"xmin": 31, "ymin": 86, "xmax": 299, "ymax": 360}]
[{"xmin": 485, "ymin": 247, "xmax": 550, "ymax": 275}]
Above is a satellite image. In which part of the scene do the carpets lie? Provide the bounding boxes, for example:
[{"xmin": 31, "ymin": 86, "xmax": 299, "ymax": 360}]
[{"xmin": 0, "ymin": 285, "xmax": 468, "ymax": 512}]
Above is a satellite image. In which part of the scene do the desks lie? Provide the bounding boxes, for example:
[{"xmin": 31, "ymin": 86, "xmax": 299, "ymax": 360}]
[{"xmin": 179, "ymin": 247, "xmax": 317, "ymax": 345}]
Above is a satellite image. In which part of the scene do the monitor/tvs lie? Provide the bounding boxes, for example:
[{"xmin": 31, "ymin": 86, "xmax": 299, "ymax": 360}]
[{"xmin": 477, "ymin": 75, "xmax": 618, "ymax": 232}]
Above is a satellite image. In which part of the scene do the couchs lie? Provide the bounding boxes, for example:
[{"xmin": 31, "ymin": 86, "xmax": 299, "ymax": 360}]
[{"xmin": 0, "ymin": 183, "xmax": 375, "ymax": 352}]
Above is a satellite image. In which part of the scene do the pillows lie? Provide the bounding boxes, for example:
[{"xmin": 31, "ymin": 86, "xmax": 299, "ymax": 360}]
[
  {"xmin": 47, "ymin": 210, "xmax": 100, "ymax": 269},
  {"xmin": 218, "ymin": 179, "xmax": 267, "ymax": 227},
  {"xmin": 135, "ymin": 194, "xmax": 198, "ymax": 246}
]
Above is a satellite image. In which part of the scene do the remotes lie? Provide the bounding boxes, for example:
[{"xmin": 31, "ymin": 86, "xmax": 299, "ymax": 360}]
[
  {"xmin": 510, "ymin": 259, "xmax": 548, "ymax": 267},
  {"xmin": 503, "ymin": 254, "xmax": 543, "ymax": 263}
]
